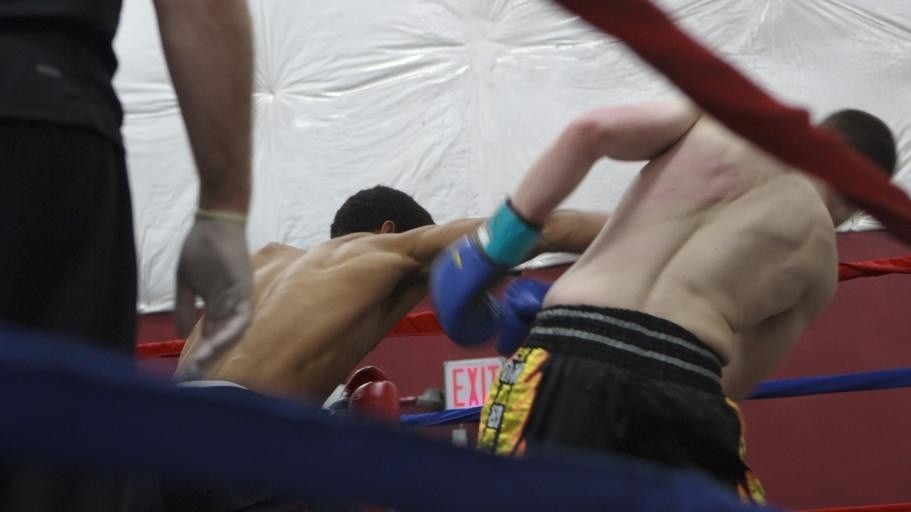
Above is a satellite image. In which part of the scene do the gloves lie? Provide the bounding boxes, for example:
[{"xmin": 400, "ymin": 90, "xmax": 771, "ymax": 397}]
[
  {"xmin": 426, "ymin": 196, "xmax": 543, "ymax": 352},
  {"xmin": 333, "ymin": 360, "xmax": 402, "ymax": 436},
  {"xmin": 174, "ymin": 208, "xmax": 258, "ymax": 371},
  {"xmin": 499, "ymin": 272, "xmax": 558, "ymax": 363}
]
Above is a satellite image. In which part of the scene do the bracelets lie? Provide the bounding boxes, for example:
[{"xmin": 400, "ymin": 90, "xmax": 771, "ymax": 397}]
[{"xmin": 189, "ymin": 207, "xmax": 252, "ymax": 227}]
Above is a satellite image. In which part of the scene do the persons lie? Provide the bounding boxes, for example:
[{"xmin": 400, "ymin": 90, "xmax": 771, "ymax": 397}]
[
  {"xmin": 430, "ymin": 91, "xmax": 898, "ymax": 510},
  {"xmin": 4, "ymin": 0, "xmax": 258, "ymax": 378},
  {"xmin": 173, "ymin": 186, "xmax": 611, "ymax": 408}
]
